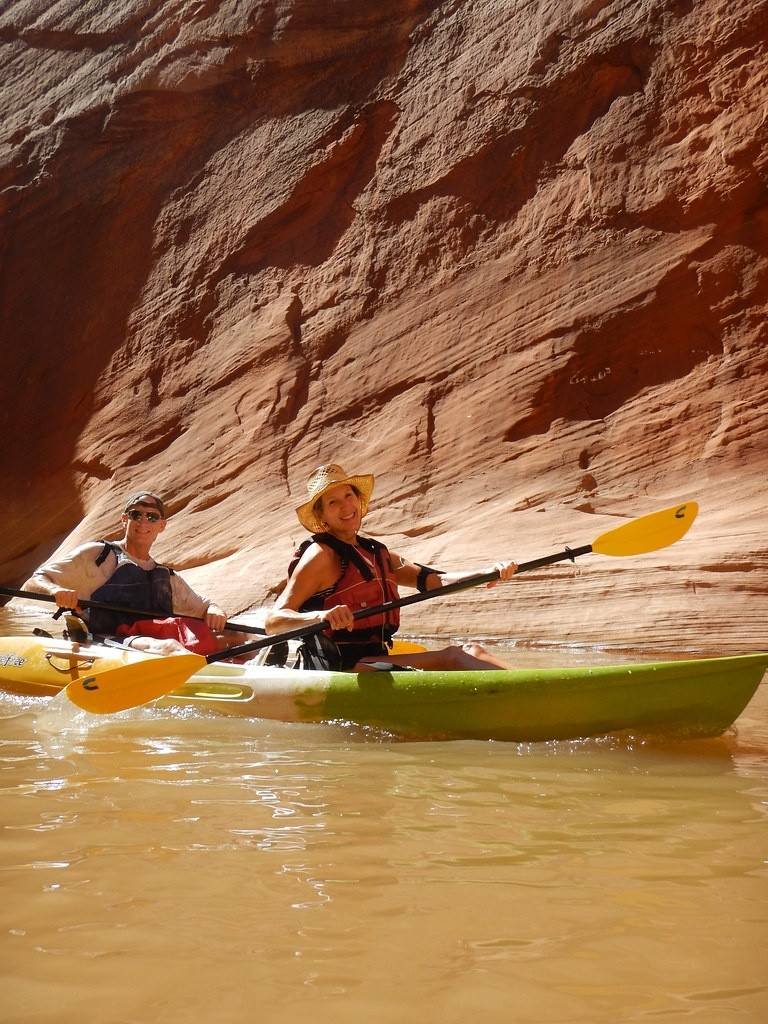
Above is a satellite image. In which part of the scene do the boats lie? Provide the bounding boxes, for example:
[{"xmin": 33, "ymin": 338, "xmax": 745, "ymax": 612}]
[{"xmin": 1, "ymin": 633, "xmax": 768, "ymax": 739}]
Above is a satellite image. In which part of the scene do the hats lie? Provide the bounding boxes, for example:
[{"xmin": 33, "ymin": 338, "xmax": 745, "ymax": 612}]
[
  {"xmin": 124, "ymin": 491, "xmax": 165, "ymax": 519},
  {"xmin": 294, "ymin": 464, "xmax": 374, "ymax": 534}
]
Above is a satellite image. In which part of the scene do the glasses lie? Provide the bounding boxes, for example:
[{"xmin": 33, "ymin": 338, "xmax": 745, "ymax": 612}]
[{"xmin": 125, "ymin": 509, "xmax": 164, "ymax": 524}]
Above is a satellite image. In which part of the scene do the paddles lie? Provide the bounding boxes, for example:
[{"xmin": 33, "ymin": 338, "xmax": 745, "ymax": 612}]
[
  {"xmin": 66, "ymin": 500, "xmax": 703, "ymax": 718},
  {"xmin": 2, "ymin": 588, "xmax": 427, "ymax": 659}
]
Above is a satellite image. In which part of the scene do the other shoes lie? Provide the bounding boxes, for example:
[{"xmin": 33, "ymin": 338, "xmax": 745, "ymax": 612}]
[{"xmin": 243, "ymin": 640, "xmax": 289, "ymax": 667}]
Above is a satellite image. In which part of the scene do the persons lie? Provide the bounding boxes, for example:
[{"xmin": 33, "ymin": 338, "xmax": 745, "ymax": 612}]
[
  {"xmin": 27, "ymin": 490, "xmax": 263, "ymax": 661},
  {"xmin": 265, "ymin": 464, "xmax": 519, "ymax": 672}
]
